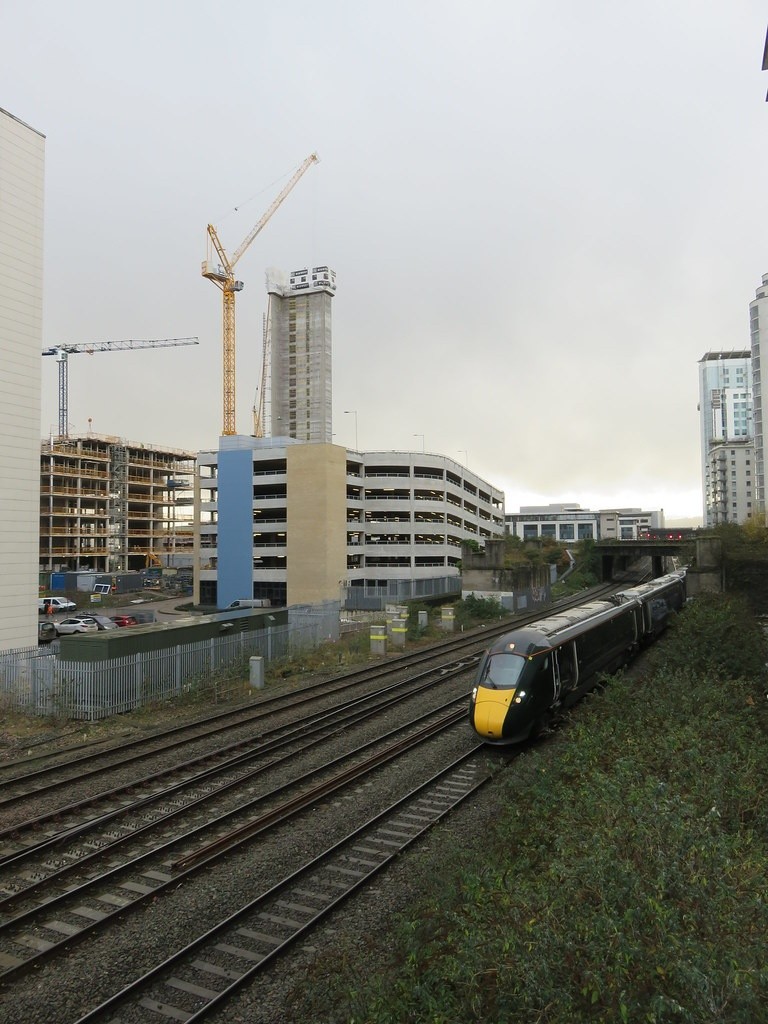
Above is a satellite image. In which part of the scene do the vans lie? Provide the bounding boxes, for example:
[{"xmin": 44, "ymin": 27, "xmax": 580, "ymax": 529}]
[{"xmin": 38, "ymin": 597, "xmax": 76, "ymax": 611}]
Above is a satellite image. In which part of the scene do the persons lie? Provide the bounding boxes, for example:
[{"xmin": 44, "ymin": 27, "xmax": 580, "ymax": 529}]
[{"xmin": 44, "ymin": 602, "xmax": 54, "ymax": 618}]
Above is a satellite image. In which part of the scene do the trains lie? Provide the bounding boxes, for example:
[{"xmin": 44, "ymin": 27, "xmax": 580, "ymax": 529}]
[{"xmin": 469, "ymin": 563, "xmax": 691, "ymax": 745}]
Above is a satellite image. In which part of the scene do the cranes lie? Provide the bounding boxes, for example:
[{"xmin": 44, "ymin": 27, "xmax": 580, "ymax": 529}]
[
  {"xmin": 42, "ymin": 337, "xmax": 199, "ymax": 441},
  {"xmin": 202, "ymin": 153, "xmax": 321, "ymax": 435}
]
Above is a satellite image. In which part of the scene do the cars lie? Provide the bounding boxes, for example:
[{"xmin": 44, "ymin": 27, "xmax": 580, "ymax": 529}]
[{"xmin": 38, "ymin": 611, "xmax": 137, "ymax": 640}]
